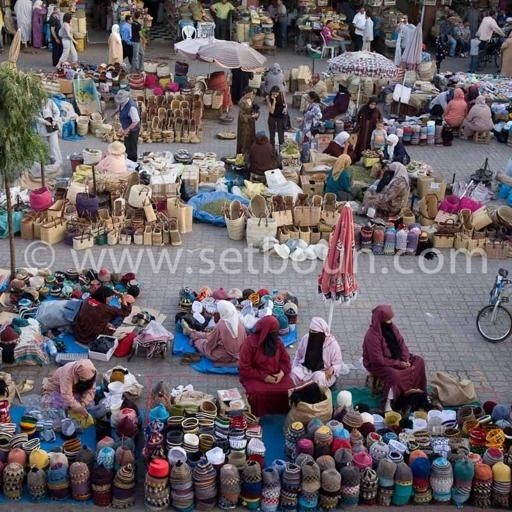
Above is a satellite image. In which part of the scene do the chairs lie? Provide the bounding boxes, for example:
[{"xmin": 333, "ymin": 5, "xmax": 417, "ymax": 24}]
[{"xmin": 320, "ymin": 31, "xmax": 338, "ymax": 59}]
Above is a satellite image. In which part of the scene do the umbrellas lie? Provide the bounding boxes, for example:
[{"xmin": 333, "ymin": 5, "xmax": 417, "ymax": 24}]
[
  {"xmin": 321, "ymin": 50, "xmax": 399, "ymax": 115},
  {"xmin": 402, "ymin": 22, "xmax": 422, "ymax": 71},
  {"xmin": 173, "ymin": 39, "xmax": 266, "ymax": 96},
  {"xmin": 2, "ymin": 28, "xmax": 21, "ymax": 73},
  {"xmin": 318, "ymin": 203, "xmax": 357, "ymax": 334}
]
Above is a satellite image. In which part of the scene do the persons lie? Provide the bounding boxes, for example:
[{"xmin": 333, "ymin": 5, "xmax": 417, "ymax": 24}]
[
  {"xmin": 352, "ymin": 6, "xmax": 366, "ymax": 52},
  {"xmin": 276, "ymin": 0, "xmax": 287, "ymax": 48},
  {"xmin": 119, "ymin": 16, "xmax": 133, "ymax": 65},
  {"xmin": 322, "ymin": 131, "xmax": 355, "ymax": 166},
  {"xmin": 267, "ymin": 0, "xmax": 278, "ymax": 23},
  {"xmin": 354, "ymin": 96, "xmax": 383, "ymax": 163},
  {"xmin": 370, "ymin": 121, "xmax": 387, "ymax": 151},
  {"xmin": 108, "ymin": 24, "xmax": 123, "ymax": 65},
  {"xmin": 114, "ymin": 89, "xmax": 141, "ymax": 162},
  {"xmin": 301, "ymin": 91, "xmax": 323, "ymax": 163},
  {"xmin": 478, "ymin": 10, "xmax": 508, "ymax": 63},
  {"xmin": 461, "ymin": 1, "xmax": 483, "ymax": 38},
  {"xmin": 260, "ymin": 63, "xmax": 286, "ymax": 102},
  {"xmin": 96, "ymin": 141, "xmax": 127, "ymax": 195},
  {"xmin": 503, "ymin": 17, "xmax": 512, "ymax": 38},
  {"xmin": 181, "ymin": 300, "xmax": 247, "ymax": 363},
  {"xmin": 131, "ymin": 11, "xmax": 147, "ymax": 73},
  {"xmin": 299, "ymin": 73, "xmax": 325, "ymax": 114},
  {"xmin": 230, "ymin": 69, "xmax": 253, "ymax": 105},
  {"xmin": 211, "ymin": 0, "xmax": 236, "ymax": 39},
  {"xmin": 497, "ymin": 9, "xmax": 507, "ymax": 27},
  {"xmin": 56, "ymin": 13, "xmax": 78, "ymax": 71},
  {"xmin": 434, "ymin": 24, "xmax": 447, "ymax": 74},
  {"xmin": 397, "ymin": 17, "xmax": 416, "ymax": 56},
  {"xmin": 325, "ymin": 154, "xmax": 364, "ymax": 202},
  {"xmin": 363, "ymin": 162, "xmax": 410, "ymax": 216},
  {"xmin": 14, "ymin": 0, "xmax": 33, "ymax": 48},
  {"xmin": 364, "ymin": 306, "xmax": 427, "ymax": 415},
  {"xmin": 42, "ymin": 358, "xmax": 96, "ymax": 417},
  {"xmin": 240, "ymin": 315, "xmax": 295, "ymax": 417},
  {"xmin": 439, "ymin": 14, "xmax": 463, "ymax": 57},
  {"xmin": 322, "ymin": 19, "xmax": 347, "ymax": 57},
  {"xmin": 320, "ymin": 78, "xmax": 351, "ymax": 122},
  {"xmin": 421, "ymin": 43, "xmax": 432, "ymax": 63},
  {"xmin": 72, "ymin": 286, "xmax": 132, "ymax": 345},
  {"xmin": 290, "ymin": 318, "xmax": 343, "ymax": 391},
  {"xmin": 35, "ymin": 98, "xmax": 62, "ymax": 166},
  {"xmin": 463, "ymin": 95, "xmax": 494, "ymax": 140},
  {"xmin": 467, "ymin": 32, "xmax": 489, "ymax": 73},
  {"xmin": 0, "ymin": 9, "xmax": 6, "ymax": 51},
  {"xmin": 237, "ymin": 87, "xmax": 261, "ymax": 160},
  {"xmin": 266, "ymin": 86, "xmax": 288, "ymax": 155},
  {"xmin": 458, "ymin": 18, "xmax": 471, "ymax": 57},
  {"xmin": 395, "ymin": 14, "xmax": 409, "ymax": 34},
  {"xmin": 362, "ymin": 9, "xmax": 374, "ymax": 52},
  {"xmin": 31, "ymin": 0, "xmax": 47, "ymax": 49},
  {"xmin": 429, "ymin": 89, "xmax": 454, "ymax": 120},
  {"xmin": 250, "ymin": 134, "xmax": 282, "ymax": 175},
  {"xmin": 4, "ymin": 7, "xmax": 16, "ymax": 36},
  {"xmin": 383, "ymin": 134, "xmax": 410, "ymax": 167},
  {"xmin": 49, "ymin": 7, "xmax": 62, "ymax": 66},
  {"xmin": 465, "ymin": 85, "xmax": 479, "ymax": 109},
  {"xmin": 0, "ymin": 377, "xmax": 11, "ymax": 424},
  {"xmin": 490, "ymin": 158, "xmax": 512, "ymax": 207},
  {"xmin": 442, "ymin": 87, "xmax": 468, "ymax": 133}
]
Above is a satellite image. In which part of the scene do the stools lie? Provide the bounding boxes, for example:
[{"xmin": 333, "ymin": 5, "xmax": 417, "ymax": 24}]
[
  {"xmin": 473, "ymin": 129, "xmax": 490, "ymax": 143},
  {"xmin": 366, "ymin": 374, "xmax": 384, "ymax": 394}
]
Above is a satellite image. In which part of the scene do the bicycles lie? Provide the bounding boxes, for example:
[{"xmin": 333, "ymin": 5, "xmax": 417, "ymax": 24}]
[
  {"xmin": 475, "ymin": 268, "xmax": 511, "ymax": 346},
  {"xmin": 476, "ymin": 34, "xmax": 501, "ymax": 71}
]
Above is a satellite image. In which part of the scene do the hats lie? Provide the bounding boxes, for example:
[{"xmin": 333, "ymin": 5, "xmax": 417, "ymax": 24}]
[
  {"xmin": 290, "ymin": 249, "xmax": 307, "ymax": 263},
  {"xmin": 286, "ymin": 239, "xmax": 297, "ymax": 251},
  {"xmin": 259, "ymin": 237, "xmax": 281, "ymax": 245},
  {"xmin": 115, "ymin": 91, "xmax": 130, "ymax": 104},
  {"xmin": 274, "ymin": 244, "xmax": 290, "ymax": 258},
  {"xmin": 296, "ymin": 239, "xmax": 308, "ymax": 249},
  {"xmin": 302, "ymin": 248, "xmax": 316, "ymax": 260},
  {"xmin": 315, "ymin": 245, "xmax": 330, "ymax": 260}
]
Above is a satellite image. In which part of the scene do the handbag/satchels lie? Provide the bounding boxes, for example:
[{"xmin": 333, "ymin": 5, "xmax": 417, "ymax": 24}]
[
  {"xmin": 420, "ymin": 215, "xmax": 435, "ymax": 226},
  {"xmin": 63, "ymin": 223, "xmax": 81, "ymax": 245},
  {"xmin": 299, "ymin": 227, "xmax": 311, "ymax": 244},
  {"xmin": 97, "ymin": 209, "xmax": 114, "ymax": 232},
  {"xmin": 73, "ymin": 208, "xmax": 90, "ymax": 223},
  {"xmin": 286, "ymin": 225, "xmax": 301, "ymax": 241},
  {"xmin": 123, "ymin": 221, "xmax": 131, "ymax": 227},
  {"xmin": 167, "ymin": 197, "xmax": 194, "ymax": 234},
  {"xmin": 144, "ymin": 226, "xmax": 153, "ymax": 247},
  {"xmin": 277, "ymin": 226, "xmax": 292, "ymax": 245},
  {"xmin": 41, "ymin": 215, "xmax": 68, "ymax": 246},
  {"xmin": 96, "ymin": 235, "xmax": 107, "ymax": 245},
  {"xmin": 12, "ymin": 194, "xmax": 28, "ymax": 212},
  {"xmin": 108, "ymin": 226, "xmax": 120, "ymax": 247},
  {"xmin": 438, "ymin": 219, "xmax": 461, "ymax": 233},
  {"xmin": 487, "ymin": 229, "xmax": 496, "ymax": 239},
  {"xmin": 325, "ymin": 192, "xmax": 337, "ymax": 212},
  {"xmin": 78, "ymin": 222, "xmax": 93, "ymax": 234},
  {"xmin": 462, "ymin": 224, "xmax": 475, "ymax": 239},
  {"xmin": 163, "ymin": 223, "xmax": 170, "ymax": 243},
  {"xmin": 487, "ymin": 239, "xmax": 508, "ymax": 260},
  {"xmin": 247, "ymin": 214, "xmax": 278, "ymax": 249},
  {"xmin": 249, "ymin": 194, "xmax": 266, "ymax": 219},
  {"xmin": 284, "ymin": 195, "xmax": 294, "ymax": 209},
  {"xmin": 129, "ymin": 183, "xmax": 154, "ymax": 207},
  {"xmin": 248, "ymin": 207, "xmax": 272, "ymax": 223},
  {"xmin": 309, "ymin": 227, "xmax": 321, "ymax": 244},
  {"xmin": 222, "ymin": 201, "xmax": 246, "ymax": 240},
  {"xmin": 22, "ymin": 210, "xmax": 44, "ymax": 241},
  {"xmin": 47, "ymin": 199, "xmax": 70, "ymax": 221},
  {"xmin": 294, "ymin": 204, "xmax": 322, "ymax": 227},
  {"xmin": 505, "ymin": 243, "xmax": 512, "ymax": 258},
  {"xmin": 98, "ymin": 224, "xmax": 104, "ymax": 235},
  {"xmin": 133, "ymin": 211, "xmax": 143, "ymax": 226},
  {"xmin": 34, "ymin": 215, "xmax": 47, "ymax": 239},
  {"xmin": 142, "ymin": 196, "xmax": 156, "ymax": 222},
  {"xmin": 417, "ymin": 194, "xmax": 440, "ymax": 217},
  {"xmin": 311, "ymin": 195, "xmax": 323, "ymax": 206},
  {"xmin": 470, "ymin": 236, "xmax": 489, "ymax": 257},
  {"xmin": 460, "ymin": 208, "xmax": 473, "ymax": 227},
  {"xmin": 136, "ymin": 93, "xmax": 205, "ymax": 144},
  {"xmin": 434, "ymin": 230, "xmax": 456, "ymax": 250},
  {"xmin": 271, "ymin": 207, "xmax": 294, "ymax": 227},
  {"xmin": 113, "ymin": 219, "xmax": 122, "ymax": 230},
  {"xmin": 435, "ymin": 210, "xmax": 457, "ymax": 225},
  {"xmin": 453, "ymin": 229, "xmax": 470, "ymax": 251},
  {"xmin": 120, "ymin": 229, "xmax": 133, "ymax": 248},
  {"xmin": 322, "ymin": 232, "xmax": 332, "ymax": 240},
  {"xmin": 169, "ymin": 217, "xmax": 184, "ymax": 247},
  {"xmin": 470, "ymin": 204, "xmax": 493, "ymax": 230},
  {"xmin": 134, "ymin": 227, "xmax": 144, "ymax": 246},
  {"xmin": 229, "ymin": 200, "xmax": 240, "ymax": 220},
  {"xmin": 90, "ymin": 227, "xmax": 100, "ymax": 237},
  {"xmin": 322, "ymin": 211, "xmax": 341, "ymax": 226},
  {"xmin": 474, "ymin": 229, "xmax": 487, "ymax": 239},
  {"xmin": 153, "ymin": 226, "xmax": 163, "ymax": 247},
  {"xmin": 319, "ymin": 222, "xmax": 334, "ymax": 233},
  {"xmin": 295, "ymin": 193, "xmax": 310, "ymax": 207},
  {"xmin": 272, "ymin": 195, "xmax": 283, "ymax": 213},
  {"xmin": 73, "ymin": 230, "xmax": 95, "ymax": 252},
  {"xmin": 65, "ymin": 202, "xmax": 79, "ymax": 220}
]
